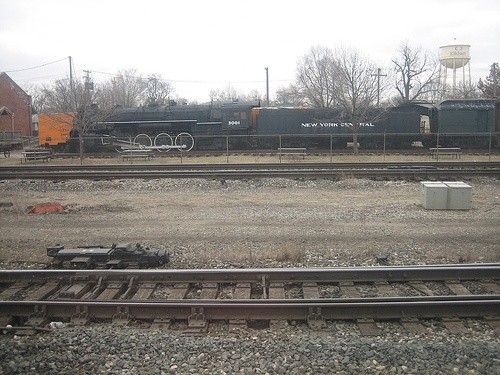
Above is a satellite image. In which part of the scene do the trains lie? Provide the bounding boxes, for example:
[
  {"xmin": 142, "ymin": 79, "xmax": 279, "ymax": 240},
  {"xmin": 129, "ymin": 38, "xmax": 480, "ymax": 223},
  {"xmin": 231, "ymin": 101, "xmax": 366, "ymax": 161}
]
[{"xmin": 66, "ymin": 100, "xmax": 499, "ymax": 154}]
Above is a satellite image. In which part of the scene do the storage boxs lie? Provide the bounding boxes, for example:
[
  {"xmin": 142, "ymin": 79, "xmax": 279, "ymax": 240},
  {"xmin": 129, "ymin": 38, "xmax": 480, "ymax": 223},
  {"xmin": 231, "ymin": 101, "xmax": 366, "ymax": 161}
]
[{"xmin": 420, "ymin": 180, "xmax": 473, "ymax": 211}]
